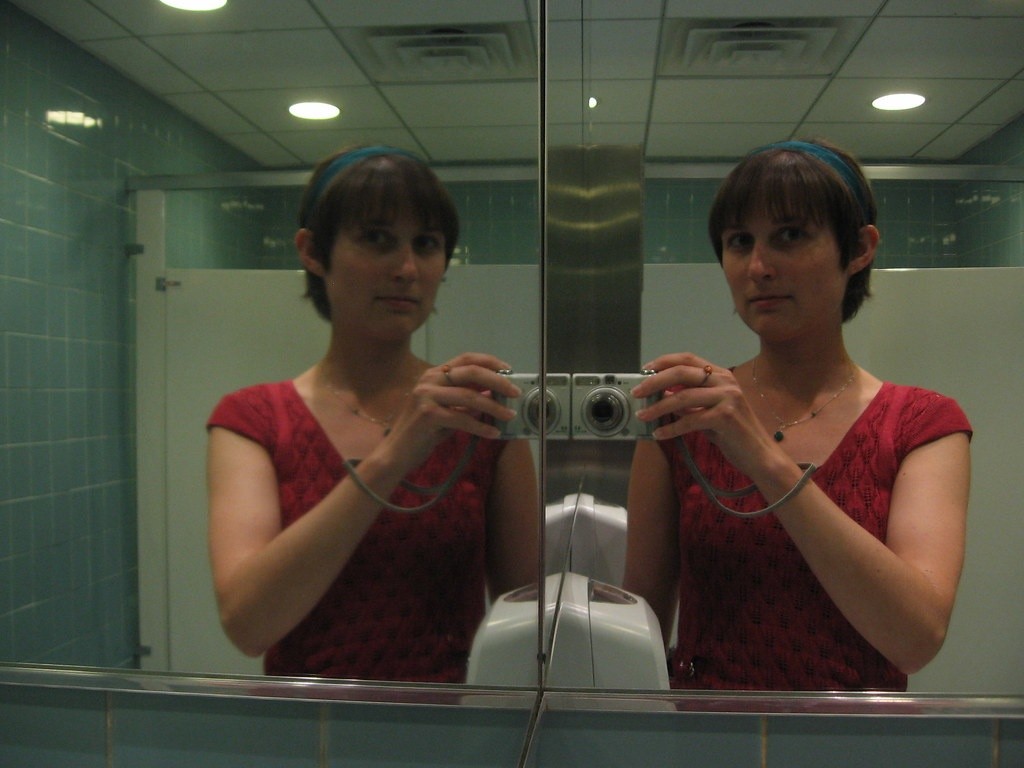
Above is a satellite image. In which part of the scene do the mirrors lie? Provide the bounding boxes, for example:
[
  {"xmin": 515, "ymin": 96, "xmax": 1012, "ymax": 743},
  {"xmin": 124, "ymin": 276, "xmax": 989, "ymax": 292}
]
[{"xmin": 2, "ymin": 1, "xmax": 1024, "ymax": 719}]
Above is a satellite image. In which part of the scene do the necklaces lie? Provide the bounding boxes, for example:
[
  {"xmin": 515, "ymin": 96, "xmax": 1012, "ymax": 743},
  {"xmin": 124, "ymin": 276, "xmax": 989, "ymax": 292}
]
[
  {"xmin": 752, "ymin": 359, "xmax": 855, "ymax": 442},
  {"xmin": 334, "ymin": 390, "xmax": 408, "ymax": 437}
]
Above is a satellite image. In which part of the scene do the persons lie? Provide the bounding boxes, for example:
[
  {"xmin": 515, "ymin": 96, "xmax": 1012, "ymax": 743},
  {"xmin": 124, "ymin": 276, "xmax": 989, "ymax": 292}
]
[
  {"xmin": 621, "ymin": 141, "xmax": 975, "ymax": 696},
  {"xmin": 207, "ymin": 144, "xmax": 540, "ymax": 684}
]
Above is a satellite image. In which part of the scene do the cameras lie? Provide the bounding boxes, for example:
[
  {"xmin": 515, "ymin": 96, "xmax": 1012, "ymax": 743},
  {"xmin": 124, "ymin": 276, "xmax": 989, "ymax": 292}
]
[
  {"xmin": 571, "ymin": 369, "xmax": 661, "ymax": 440},
  {"xmin": 493, "ymin": 370, "xmax": 572, "ymax": 439}
]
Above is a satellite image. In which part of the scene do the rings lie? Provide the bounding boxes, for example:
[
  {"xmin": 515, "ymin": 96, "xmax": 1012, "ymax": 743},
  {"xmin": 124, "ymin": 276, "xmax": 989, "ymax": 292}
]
[
  {"xmin": 702, "ymin": 366, "xmax": 713, "ymax": 386},
  {"xmin": 443, "ymin": 365, "xmax": 453, "ymax": 384}
]
